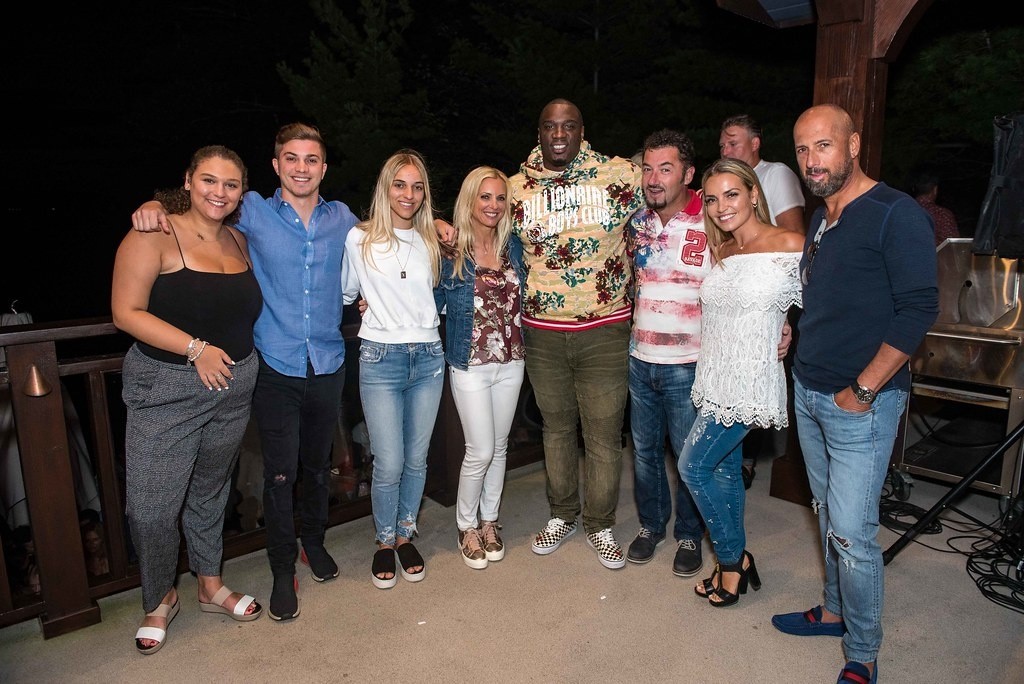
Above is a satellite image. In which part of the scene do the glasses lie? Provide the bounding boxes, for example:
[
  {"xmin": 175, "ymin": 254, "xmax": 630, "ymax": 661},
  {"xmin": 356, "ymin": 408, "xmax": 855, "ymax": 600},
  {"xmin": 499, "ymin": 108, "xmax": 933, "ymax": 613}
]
[{"xmin": 800, "ymin": 241, "xmax": 821, "ymax": 286}]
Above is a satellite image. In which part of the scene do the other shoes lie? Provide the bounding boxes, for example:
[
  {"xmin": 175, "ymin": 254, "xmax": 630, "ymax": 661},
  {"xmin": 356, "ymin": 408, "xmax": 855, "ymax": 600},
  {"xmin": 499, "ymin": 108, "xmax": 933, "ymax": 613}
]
[
  {"xmin": 836, "ymin": 658, "xmax": 877, "ymax": 684},
  {"xmin": 772, "ymin": 605, "xmax": 848, "ymax": 637},
  {"xmin": 742, "ymin": 467, "xmax": 755, "ymax": 492}
]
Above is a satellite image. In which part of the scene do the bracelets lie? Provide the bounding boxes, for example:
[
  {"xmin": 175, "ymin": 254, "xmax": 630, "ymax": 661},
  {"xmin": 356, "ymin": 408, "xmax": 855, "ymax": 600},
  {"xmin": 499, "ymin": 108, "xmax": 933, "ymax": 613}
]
[{"xmin": 184, "ymin": 337, "xmax": 210, "ymax": 369}]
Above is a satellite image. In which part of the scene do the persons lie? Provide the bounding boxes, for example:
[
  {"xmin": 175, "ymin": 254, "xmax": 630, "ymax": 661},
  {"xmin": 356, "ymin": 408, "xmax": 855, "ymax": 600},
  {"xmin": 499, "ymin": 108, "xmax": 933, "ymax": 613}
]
[
  {"xmin": 340, "ymin": 148, "xmax": 445, "ymax": 588},
  {"xmin": 358, "ymin": 167, "xmax": 526, "ymax": 569},
  {"xmin": 113, "ymin": 146, "xmax": 264, "ymax": 654},
  {"xmin": 505, "ymin": 98, "xmax": 650, "ymax": 570},
  {"xmin": 769, "ymin": 104, "xmax": 941, "ymax": 684},
  {"xmin": 719, "ymin": 115, "xmax": 808, "ymax": 492},
  {"xmin": 623, "ymin": 129, "xmax": 791, "ymax": 577},
  {"xmin": 903, "ymin": 164, "xmax": 961, "ymax": 247},
  {"xmin": 676, "ymin": 159, "xmax": 806, "ymax": 607},
  {"xmin": 131, "ymin": 124, "xmax": 457, "ymax": 622}
]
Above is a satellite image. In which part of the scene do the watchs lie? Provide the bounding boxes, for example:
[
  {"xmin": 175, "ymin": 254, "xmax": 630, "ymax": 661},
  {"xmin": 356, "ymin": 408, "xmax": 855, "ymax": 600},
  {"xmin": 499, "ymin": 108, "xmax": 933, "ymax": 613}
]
[{"xmin": 851, "ymin": 378, "xmax": 875, "ymax": 405}]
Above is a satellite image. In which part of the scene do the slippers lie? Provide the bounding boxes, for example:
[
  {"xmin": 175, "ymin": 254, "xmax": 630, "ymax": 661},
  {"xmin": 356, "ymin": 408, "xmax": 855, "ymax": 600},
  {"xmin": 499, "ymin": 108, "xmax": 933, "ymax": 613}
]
[
  {"xmin": 199, "ymin": 587, "xmax": 262, "ymax": 622},
  {"xmin": 395, "ymin": 543, "xmax": 427, "ymax": 582},
  {"xmin": 134, "ymin": 597, "xmax": 180, "ymax": 655},
  {"xmin": 371, "ymin": 548, "xmax": 397, "ymax": 590}
]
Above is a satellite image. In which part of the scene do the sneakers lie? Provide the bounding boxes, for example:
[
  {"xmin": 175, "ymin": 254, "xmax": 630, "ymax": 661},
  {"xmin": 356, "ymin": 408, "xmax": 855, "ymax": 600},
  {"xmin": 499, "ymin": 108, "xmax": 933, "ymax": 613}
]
[
  {"xmin": 268, "ymin": 568, "xmax": 300, "ymax": 621},
  {"xmin": 531, "ymin": 515, "xmax": 578, "ymax": 554},
  {"xmin": 586, "ymin": 527, "xmax": 625, "ymax": 569},
  {"xmin": 672, "ymin": 539, "xmax": 702, "ymax": 576},
  {"xmin": 303, "ymin": 540, "xmax": 339, "ymax": 582},
  {"xmin": 627, "ymin": 526, "xmax": 666, "ymax": 563},
  {"xmin": 457, "ymin": 529, "xmax": 487, "ymax": 569},
  {"xmin": 478, "ymin": 519, "xmax": 505, "ymax": 561}
]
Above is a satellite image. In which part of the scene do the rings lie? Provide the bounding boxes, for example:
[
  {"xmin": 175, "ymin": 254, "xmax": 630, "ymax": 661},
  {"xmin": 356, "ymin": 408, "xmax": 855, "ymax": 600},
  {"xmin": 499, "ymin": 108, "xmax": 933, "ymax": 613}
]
[{"xmin": 215, "ymin": 373, "xmax": 223, "ymax": 379}]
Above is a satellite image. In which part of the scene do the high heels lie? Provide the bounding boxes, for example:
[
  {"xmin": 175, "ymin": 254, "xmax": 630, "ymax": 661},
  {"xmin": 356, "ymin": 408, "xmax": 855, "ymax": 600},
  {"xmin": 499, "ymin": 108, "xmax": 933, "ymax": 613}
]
[
  {"xmin": 695, "ymin": 563, "xmax": 749, "ymax": 598},
  {"xmin": 707, "ymin": 549, "xmax": 761, "ymax": 607}
]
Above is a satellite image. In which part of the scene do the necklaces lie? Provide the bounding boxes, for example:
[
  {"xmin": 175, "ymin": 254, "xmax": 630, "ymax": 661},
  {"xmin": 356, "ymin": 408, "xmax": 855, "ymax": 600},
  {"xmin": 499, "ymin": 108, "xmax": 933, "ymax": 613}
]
[
  {"xmin": 738, "ymin": 223, "xmax": 765, "ymax": 249},
  {"xmin": 388, "ymin": 230, "xmax": 414, "ymax": 278},
  {"xmin": 482, "ymin": 250, "xmax": 488, "ymax": 254}
]
